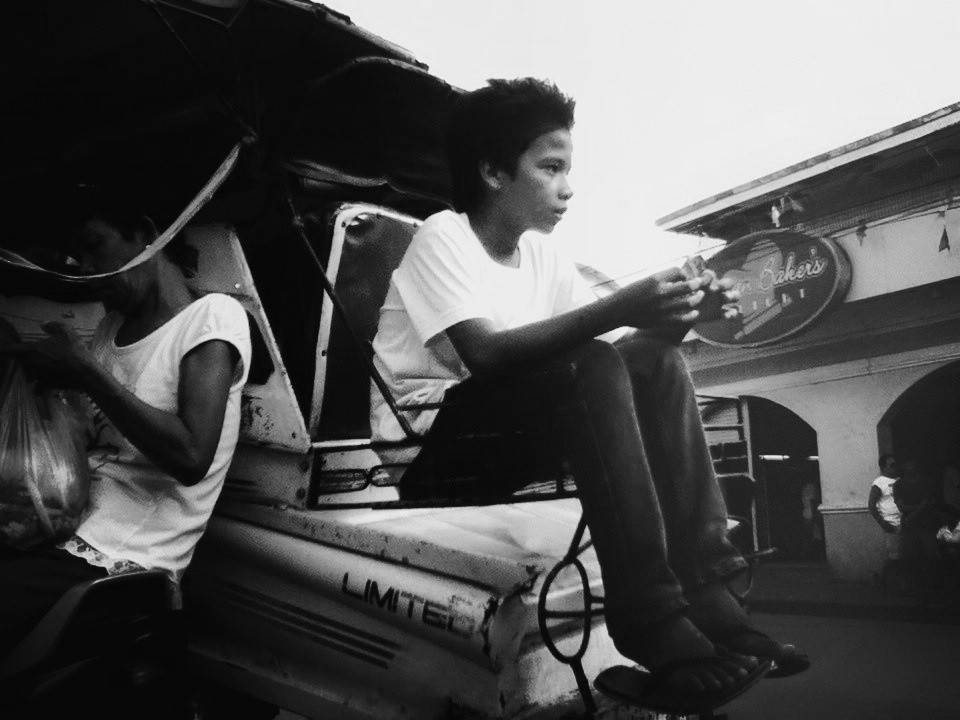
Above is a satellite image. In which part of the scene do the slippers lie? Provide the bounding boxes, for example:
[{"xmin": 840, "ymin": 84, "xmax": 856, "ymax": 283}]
[
  {"xmin": 593, "ymin": 641, "xmax": 768, "ymax": 714},
  {"xmin": 705, "ymin": 621, "xmax": 809, "ymax": 678}
]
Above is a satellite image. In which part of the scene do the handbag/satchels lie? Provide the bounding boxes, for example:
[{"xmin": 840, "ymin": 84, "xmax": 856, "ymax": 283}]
[{"xmin": 0, "ymin": 354, "xmax": 90, "ymax": 552}]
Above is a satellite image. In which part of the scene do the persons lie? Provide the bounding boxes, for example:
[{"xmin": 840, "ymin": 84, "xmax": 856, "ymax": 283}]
[
  {"xmin": 801, "ymin": 473, "xmax": 822, "ymax": 565},
  {"xmin": 370, "ymin": 77, "xmax": 813, "ymax": 720},
  {"xmin": 0, "ymin": 168, "xmax": 256, "ymax": 720},
  {"xmin": 868, "ymin": 452, "xmax": 945, "ymax": 604}
]
